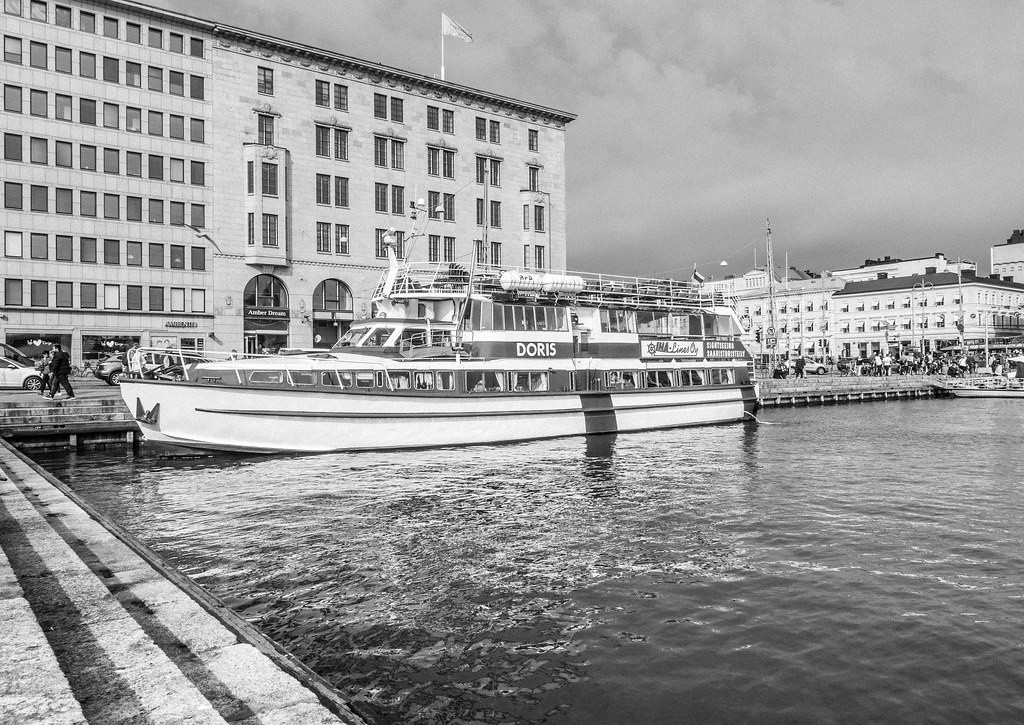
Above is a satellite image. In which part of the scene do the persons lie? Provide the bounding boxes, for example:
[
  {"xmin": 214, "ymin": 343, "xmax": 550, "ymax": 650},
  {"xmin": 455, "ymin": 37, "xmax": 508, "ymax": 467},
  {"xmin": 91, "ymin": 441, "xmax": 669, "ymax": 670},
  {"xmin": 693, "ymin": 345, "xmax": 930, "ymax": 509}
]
[
  {"xmin": 225, "ymin": 349, "xmax": 238, "ymax": 361},
  {"xmin": 35, "ymin": 345, "xmax": 76, "ymax": 402},
  {"xmin": 773, "ymin": 350, "xmax": 1024, "ymax": 377},
  {"xmin": 122, "ymin": 340, "xmax": 178, "ymax": 382}
]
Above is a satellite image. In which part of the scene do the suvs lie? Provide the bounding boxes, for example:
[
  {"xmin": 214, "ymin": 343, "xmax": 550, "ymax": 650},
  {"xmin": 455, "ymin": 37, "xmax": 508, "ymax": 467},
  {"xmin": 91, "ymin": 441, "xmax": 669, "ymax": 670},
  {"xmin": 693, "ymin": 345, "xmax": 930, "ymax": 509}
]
[{"xmin": 836, "ymin": 357, "xmax": 872, "ymax": 374}]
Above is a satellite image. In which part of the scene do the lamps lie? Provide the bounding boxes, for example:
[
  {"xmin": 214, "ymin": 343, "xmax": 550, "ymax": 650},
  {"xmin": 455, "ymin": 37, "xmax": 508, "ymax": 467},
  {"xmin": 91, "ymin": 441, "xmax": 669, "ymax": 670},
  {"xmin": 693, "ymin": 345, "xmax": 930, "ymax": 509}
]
[{"xmin": 226, "ymin": 297, "xmax": 231, "ymax": 305}]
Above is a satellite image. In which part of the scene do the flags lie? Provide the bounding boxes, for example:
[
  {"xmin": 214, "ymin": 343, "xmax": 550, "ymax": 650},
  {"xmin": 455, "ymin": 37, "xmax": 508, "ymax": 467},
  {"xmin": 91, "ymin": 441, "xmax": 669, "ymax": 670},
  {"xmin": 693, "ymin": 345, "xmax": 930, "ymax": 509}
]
[{"xmin": 441, "ymin": 13, "xmax": 474, "ymax": 43}]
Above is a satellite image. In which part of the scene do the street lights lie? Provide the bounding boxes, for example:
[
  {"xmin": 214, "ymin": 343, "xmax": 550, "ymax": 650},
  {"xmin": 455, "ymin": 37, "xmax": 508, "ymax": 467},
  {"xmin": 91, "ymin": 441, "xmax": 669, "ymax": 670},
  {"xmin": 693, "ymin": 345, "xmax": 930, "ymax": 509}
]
[
  {"xmin": 944, "ymin": 256, "xmax": 975, "ymax": 354},
  {"xmin": 911, "ymin": 279, "xmax": 934, "ymax": 353},
  {"xmin": 810, "ymin": 271, "xmax": 837, "ymax": 363}
]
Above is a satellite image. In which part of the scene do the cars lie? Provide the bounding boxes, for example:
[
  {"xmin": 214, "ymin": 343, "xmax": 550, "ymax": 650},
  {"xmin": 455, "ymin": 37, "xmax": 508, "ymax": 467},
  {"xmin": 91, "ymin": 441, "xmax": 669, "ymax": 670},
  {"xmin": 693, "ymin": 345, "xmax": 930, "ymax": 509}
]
[
  {"xmin": 0, "ymin": 356, "xmax": 49, "ymax": 391},
  {"xmin": 95, "ymin": 353, "xmax": 122, "ymax": 386},
  {"xmin": 785, "ymin": 356, "xmax": 829, "ymax": 375},
  {"xmin": 892, "ymin": 359, "xmax": 901, "ymax": 373},
  {"xmin": 0, "ymin": 342, "xmax": 39, "ymax": 367}
]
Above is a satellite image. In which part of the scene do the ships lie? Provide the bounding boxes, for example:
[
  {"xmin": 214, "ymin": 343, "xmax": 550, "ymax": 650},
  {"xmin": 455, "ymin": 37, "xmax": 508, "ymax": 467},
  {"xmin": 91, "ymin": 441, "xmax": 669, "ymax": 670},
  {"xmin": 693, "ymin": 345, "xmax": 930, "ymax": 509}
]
[{"xmin": 120, "ymin": 169, "xmax": 765, "ymax": 453}]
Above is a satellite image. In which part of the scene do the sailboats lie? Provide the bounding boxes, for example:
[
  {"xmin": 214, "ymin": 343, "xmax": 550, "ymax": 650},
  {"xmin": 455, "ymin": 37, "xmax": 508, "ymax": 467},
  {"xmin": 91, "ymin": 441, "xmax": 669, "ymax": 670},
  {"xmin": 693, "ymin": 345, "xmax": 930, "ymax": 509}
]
[{"xmin": 942, "ymin": 310, "xmax": 1024, "ymax": 399}]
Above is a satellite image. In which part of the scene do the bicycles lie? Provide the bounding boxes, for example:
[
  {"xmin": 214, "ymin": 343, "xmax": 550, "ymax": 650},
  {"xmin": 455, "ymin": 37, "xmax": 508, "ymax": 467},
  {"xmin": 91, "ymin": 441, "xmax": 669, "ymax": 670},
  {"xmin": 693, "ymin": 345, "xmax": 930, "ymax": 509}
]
[{"xmin": 70, "ymin": 363, "xmax": 81, "ymax": 376}]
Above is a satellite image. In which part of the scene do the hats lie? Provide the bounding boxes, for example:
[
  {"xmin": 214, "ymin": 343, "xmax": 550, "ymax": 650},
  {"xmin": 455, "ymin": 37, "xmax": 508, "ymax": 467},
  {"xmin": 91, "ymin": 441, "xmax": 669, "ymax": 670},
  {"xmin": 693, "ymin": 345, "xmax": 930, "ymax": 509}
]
[{"xmin": 133, "ymin": 343, "xmax": 139, "ymax": 347}]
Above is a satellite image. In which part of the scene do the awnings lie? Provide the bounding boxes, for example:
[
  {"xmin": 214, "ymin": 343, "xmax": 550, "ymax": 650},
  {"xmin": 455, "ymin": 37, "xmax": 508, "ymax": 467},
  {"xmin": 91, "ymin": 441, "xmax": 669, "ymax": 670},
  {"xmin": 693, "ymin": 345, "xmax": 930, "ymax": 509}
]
[{"xmin": 1007, "ymin": 356, "xmax": 1024, "ymax": 363}]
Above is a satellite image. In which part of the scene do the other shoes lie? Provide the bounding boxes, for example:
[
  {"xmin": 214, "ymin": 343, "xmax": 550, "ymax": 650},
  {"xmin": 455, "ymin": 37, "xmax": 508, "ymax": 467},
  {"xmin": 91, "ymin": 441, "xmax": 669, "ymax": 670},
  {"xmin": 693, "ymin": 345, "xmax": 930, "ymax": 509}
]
[
  {"xmin": 39, "ymin": 391, "xmax": 44, "ymax": 395},
  {"xmin": 43, "ymin": 393, "xmax": 54, "ymax": 399},
  {"xmin": 63, "ymin": 394, "xmax": 75, "ymax": 401}
]
